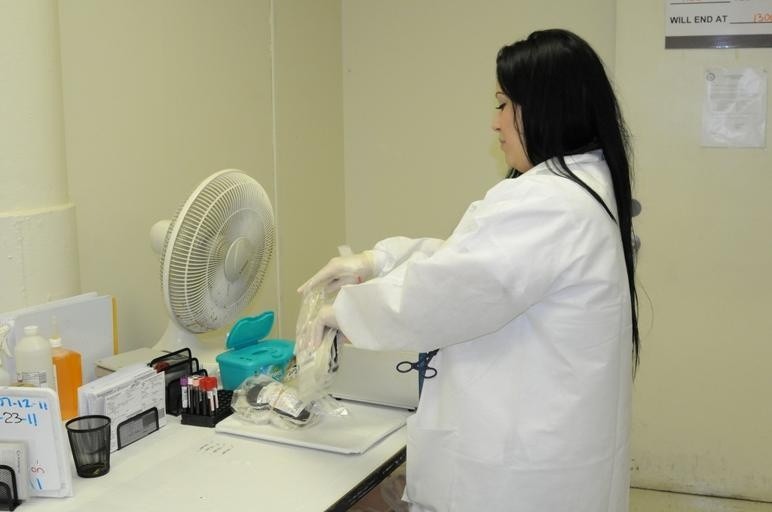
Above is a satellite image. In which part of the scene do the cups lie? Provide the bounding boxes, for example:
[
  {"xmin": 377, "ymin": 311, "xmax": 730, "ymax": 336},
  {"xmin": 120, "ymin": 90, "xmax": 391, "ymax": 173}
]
[{"xmin": 65, "ymin": 415, "xmax": 112, "ymax": 477}]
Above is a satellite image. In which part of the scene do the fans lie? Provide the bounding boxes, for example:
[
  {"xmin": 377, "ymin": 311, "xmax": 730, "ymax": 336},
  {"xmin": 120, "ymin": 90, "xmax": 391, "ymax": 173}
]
[{"xmin": 146, "ymin": 168, "xmax": 278, "ymax": 374}]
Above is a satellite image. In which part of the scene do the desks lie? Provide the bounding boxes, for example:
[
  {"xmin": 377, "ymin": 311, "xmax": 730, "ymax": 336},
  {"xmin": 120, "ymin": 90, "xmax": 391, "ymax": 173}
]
[{"xmin": 14, "ymin": 393, "xmax": 409, "ymax": 512}]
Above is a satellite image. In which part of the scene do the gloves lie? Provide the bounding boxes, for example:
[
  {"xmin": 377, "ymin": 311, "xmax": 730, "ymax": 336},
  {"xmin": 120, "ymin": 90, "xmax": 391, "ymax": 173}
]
[
  {"xmin": 309, "ymin": 304, "xmax": 350, "ymax": 346},
  {"xmin": 297, "ymin": 252, "xmax": 373, "ymax": 296}
]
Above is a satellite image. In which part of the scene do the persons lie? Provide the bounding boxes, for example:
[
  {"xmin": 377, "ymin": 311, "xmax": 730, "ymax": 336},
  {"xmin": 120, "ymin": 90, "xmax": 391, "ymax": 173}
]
[{"xmin": 296, "ymin": 27, "xmax": 638, "ymax": 511}]
